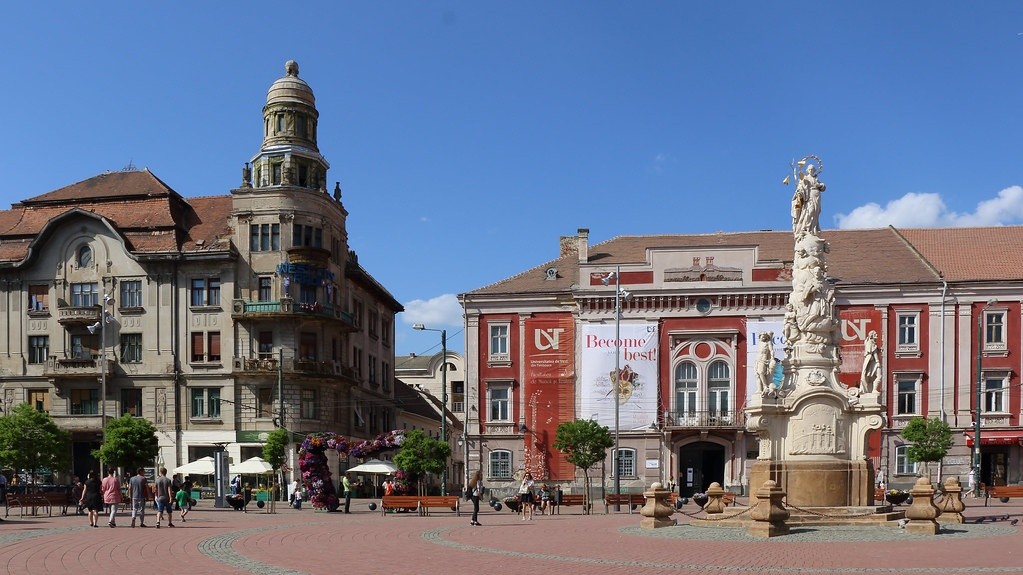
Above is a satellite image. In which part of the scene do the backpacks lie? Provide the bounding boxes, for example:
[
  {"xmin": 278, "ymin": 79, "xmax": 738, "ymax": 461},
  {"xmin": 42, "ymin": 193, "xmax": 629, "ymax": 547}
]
[
  {"xmin": 541, "ymin": 490, "xmax": 549, "ymax": 500},
  {"xmin": 463, "ymin": 485, "xmax": 476, "ymax": 502}
]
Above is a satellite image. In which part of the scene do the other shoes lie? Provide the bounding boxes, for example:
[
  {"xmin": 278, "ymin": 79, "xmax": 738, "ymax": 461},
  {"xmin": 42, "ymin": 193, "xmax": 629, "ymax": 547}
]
[
  {"xmin": 521, "ymin": 516, "xmax": 526, "ymax": 520},
  {"xmin": 108, "ymin": 522, "xmax": 115, "ymax": 527},
  {"xmin": 167, "ymin": 523, "xmax": 175, "ymax": 528},
  {"xmin": 156, "ymin": 522, "xmax": 160, "ymax": 527},
  {"xmin": 181, "ymin": 518, "xmax": 185, "ymax": 521},
  {"xmin": 131, "ymin": 517, "xmax": 135, "ymax": 527},
  {"xmin": 90, "ymin": 521, "xmax": 93, "ymax": 525},
  {"xmin": 181, "ymin": 515, "xmax": 184, "ymax": 518},
  {"xmin": 964, "ymin": 493, "xmax": 967, "ymax": 498},
  {"xmin": 469, "ymin": 520, "xmax": 473, "ymax": 526},
  {"xmin": 139, "ymin": 524, "xmax": 146, "ymax": 527},
  {"xmin": 93, "ymin": 524, "xmax": 98, "ymax": 527},
  {"xmin": 473, "ymin": 521, "xmax": 481, "ymax": 526},
  {"xmin": 527, "ymin": 516, "xmax": 532, "ymax": 520}
]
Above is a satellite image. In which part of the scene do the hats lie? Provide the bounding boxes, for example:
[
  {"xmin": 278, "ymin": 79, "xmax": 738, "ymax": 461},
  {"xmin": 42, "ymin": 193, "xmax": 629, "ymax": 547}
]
[{"xmin": 541, "ymin": 483, "xmax": 545, "ymax": 488}]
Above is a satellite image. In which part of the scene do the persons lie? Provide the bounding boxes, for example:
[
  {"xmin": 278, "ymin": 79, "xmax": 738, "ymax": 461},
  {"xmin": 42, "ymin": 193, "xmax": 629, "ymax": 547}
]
[
  {"xmin": 289, "ymin": 479, "xmax": 303, "ymax": 510},
  {"xmin": 73, "ymin": 467, "xmax": 175, "ymax": 528},
  {"xmin": 172, "ymin": 475, "xmax": 193, "ymax": 522},
  {"xmin": 235, "ymin": 474, "xmax": 240, "ymax": 487},
  {"xmin": 781, "ymin": 164, "xmax": 843, "ymax": 345},
  {"xmin": 754, "ymin": 331, "xmax": 777, "ymax": 397},
  {"xmin": 470, "ymin": 471, "xmax": 483, "ymax": 525},
  {"xmin": 859, "ymin": 330, "xmax": 884, "ymax": 393},
  {"xmin": 383, "ymin": 477, "xmax": 401, "ymax": 512},
  {"xmin": 537, "ymin": 484, "xmax": 551, "ymax": 516},
  {"xmin": 342, "ymin": 473, "xmax": 372, "ymax": 513},
  {"xmin": 521, "ymin": 473, "xmax": 534, "ymax": 520},
  {"xmin": 965, "ymin": 467, "xmax": 976, "ymax": 498},
  {"xmin": 0, "ymin": 470, "xmax": 22, "ymax": 488},
  {"xmin": 876, "ymin": 468, "xmax": 883, "ymax": 488},
  {"xmin": 668, "ymin": 477, "xmax": 676, "ymax": 492}
]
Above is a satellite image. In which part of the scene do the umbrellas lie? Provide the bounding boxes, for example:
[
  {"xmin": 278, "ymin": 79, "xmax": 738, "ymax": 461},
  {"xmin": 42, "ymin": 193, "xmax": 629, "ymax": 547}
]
[
  {"xmin": 347, "ymin": 459, "xmax": 399, "ymax": 497},
  {"xmin": 229, "ymin": 456, "xmax": 281, "ymax": 490},
  {"xmin": 173, "ymin": 456, "xmax": 215, "ymax": 482}
]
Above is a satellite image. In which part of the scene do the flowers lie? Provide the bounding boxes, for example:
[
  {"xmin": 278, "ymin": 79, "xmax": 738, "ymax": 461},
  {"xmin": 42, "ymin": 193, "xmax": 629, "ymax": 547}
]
[
  {"xmin": 879, "ymin": 489, "xmax": 910, "ymax": 497},
  {"xmin": 298, "ymin": 429, "xmax": 417, "ymax": 511},
  {"xmin": 503, "ymin": 497, "xmax": 518, "ymax": 502},
  {"xmin": 692, "ymin": 492, "xmax": 707, "ymax": 499},
  {"xmin": 225, "ymin": 493, "xmax": 244, "ymax": 500}
]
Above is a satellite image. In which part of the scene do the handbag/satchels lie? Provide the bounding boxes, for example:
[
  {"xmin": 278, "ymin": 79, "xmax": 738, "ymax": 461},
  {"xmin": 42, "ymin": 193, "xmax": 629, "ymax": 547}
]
[
  {"xmin": 879, "ymin": 482, "xmax": 884, "ymax": 490},
  {"xmin": 518, "ymin": 484, "xmax": 529, "ymax": 494}
]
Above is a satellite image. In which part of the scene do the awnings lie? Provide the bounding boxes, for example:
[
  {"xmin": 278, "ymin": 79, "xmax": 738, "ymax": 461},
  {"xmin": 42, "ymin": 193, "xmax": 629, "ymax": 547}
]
[{"xmin": 966, "ymin": 431, "xmax": 1023, "ymax": 446}]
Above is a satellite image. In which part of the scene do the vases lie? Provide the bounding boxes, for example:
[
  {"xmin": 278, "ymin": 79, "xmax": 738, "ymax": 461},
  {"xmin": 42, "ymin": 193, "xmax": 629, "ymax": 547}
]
[
  {"xmin": 693, "ymin": 498, "xmax": 709, "ymax": 508},
  {"xmin": 886, "ymin": 495, "xmax": 909, "ymax": 506},
  {"xmin": 504, "ymin": 502, "xmax": 522, "ymax": 512},
  {"xmin": 226, "ymin": 499, "xmax": 244, "ymax": 511}
]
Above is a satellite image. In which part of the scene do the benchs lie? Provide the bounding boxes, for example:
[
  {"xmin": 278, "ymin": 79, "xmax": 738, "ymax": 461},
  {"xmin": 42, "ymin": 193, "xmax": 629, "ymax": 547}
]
[
  {"xmin": 380, "ymin": 496, "xmax": 459, "ymax": 517},
  {"xmin": 605, "ymin": 493, "xmax": 678, "ymax": 514},
  {"xmin": 5, "ymin": 493, "xmax": 52, "ymax": 518},
  {"xmin": 517, "ymin": 495, "xmax": 586, "ymax": 515},
  {"xmin": 101, "ymin": 498, "xmax": 130, "ymax": 512},
  {"xmin": 45, "ymin": 493, "xmax": 78, "ymax": 517},
  {"xmin": 873, "ymin": 489, "xmax": 885, "ymax": 505},
  {"xmin": 985, "ymin": 486, "xmax": 1023, "ymax": 507}
]
